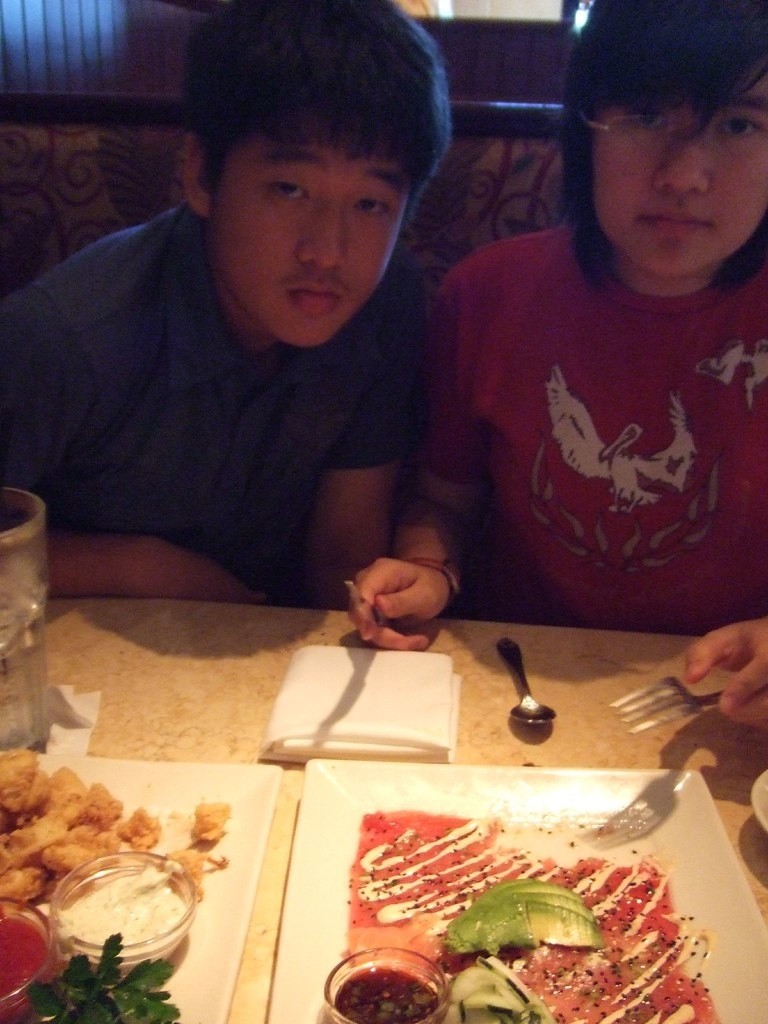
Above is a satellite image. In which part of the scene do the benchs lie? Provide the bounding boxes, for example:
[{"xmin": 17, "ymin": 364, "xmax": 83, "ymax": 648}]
[{"xmin": 0, "ymin": 94, "xmax": 568, "ymax": 319}]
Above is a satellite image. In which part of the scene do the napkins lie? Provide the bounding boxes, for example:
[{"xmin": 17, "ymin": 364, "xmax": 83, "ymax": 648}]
[
  {"xmin": 36, "ymin": 684, "xmax": 102, "ymax": 758},
  {"xmin": 258, "ymin": 645, "xmax": 463, "ymax": 764}
]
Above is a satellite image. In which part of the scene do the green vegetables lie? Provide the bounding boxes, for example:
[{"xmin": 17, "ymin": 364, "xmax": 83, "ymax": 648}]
[{"xmin": 28, "ymin": 932, "xmax": 181, "ymax": 1024}]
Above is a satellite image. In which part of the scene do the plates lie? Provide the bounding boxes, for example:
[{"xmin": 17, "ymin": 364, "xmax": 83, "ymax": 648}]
[
  {"xmin": 263, "ymin": 758, "xmax": 768, "ymax": 1024},
  {"xmin": 750, "ymin": 769, "xmax": 768, "ymax": 831},
  {"xmin": 9, "ymin": 747, "xmax": 283, "ymax": 1024}
]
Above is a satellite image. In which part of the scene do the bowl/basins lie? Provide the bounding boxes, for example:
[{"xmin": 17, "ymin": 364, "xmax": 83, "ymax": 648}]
[
  {"xmin": 0, "ymin": 896, "xmax": 61, "ymax": 1024},
  {"xmin": 51, "ymin": 852, "xmax": 196, "ymax": 975}
]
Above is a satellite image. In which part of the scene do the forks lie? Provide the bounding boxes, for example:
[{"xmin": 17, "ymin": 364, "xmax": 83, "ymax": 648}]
[{"xmin": 610, "ymin": 677, "xmax": 724, "ymax": 735}]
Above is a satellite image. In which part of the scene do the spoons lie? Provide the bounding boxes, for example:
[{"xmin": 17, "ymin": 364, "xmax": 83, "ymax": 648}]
[{"xmin": 494, "ymin": 636, "xmax": 557, "ymax": 726}]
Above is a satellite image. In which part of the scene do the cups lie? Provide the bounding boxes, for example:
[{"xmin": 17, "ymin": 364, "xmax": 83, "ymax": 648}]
[{"xmin": 0, "ymin": 486, "xmax": 49, "ymax": 755}]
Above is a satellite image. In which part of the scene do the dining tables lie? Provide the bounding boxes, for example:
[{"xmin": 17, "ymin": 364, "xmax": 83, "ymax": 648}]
[{"xmin": 0, "ymin": 596, "xmax": 768, "ymax": 1024}]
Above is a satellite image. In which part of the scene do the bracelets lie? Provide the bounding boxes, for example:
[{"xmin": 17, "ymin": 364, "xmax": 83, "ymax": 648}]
[{"xmin": 407, "ymin": 557, "xmax": 459, "ymax": 610}]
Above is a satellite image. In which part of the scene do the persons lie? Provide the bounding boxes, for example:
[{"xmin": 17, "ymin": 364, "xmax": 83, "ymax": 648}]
[
  {"xmin": 346, "ymin": 0, "xmax": 768, "ymax": 734},
  {"xmin": 0, "ymin": 0, "xmax": 453, "ymax": 612}
]
